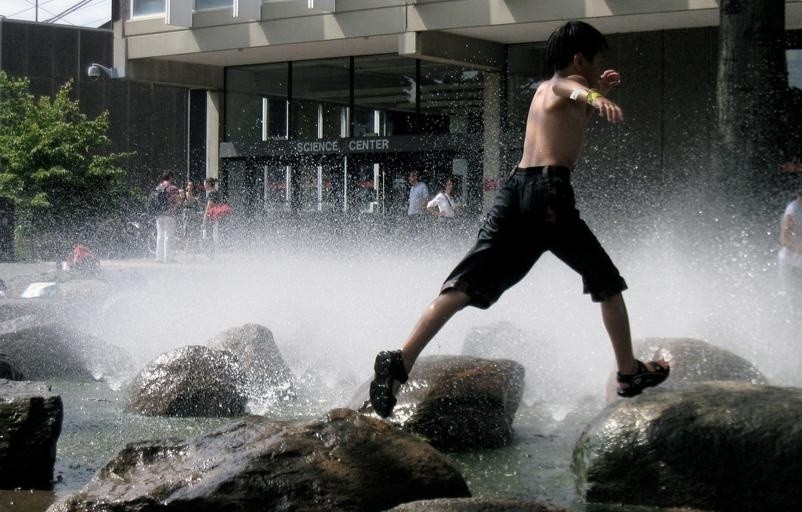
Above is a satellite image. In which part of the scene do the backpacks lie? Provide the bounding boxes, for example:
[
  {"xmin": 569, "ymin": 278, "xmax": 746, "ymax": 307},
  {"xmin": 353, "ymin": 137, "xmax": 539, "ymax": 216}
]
[{"xmin": 148, "ymin": 185, "xmax": 173, "ymax": 215}]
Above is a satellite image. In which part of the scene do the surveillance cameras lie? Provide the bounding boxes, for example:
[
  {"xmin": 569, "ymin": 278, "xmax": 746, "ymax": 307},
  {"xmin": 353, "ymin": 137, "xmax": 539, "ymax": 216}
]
[{"xmin": 87, "ymin": 65, "xmax": 101, "ymax": 81}]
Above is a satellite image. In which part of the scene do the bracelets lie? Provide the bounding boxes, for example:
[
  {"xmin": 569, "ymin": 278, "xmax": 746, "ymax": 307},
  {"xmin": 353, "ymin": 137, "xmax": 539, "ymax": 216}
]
[{"xmin": 586, "ymin": 90, "xmax": 603, "ymax": 106}]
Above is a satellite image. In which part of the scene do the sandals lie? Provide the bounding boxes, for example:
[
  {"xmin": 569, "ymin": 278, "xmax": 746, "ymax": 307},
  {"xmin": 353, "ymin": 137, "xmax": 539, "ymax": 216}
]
[
  {"xmin": 370, "ymin": 351, "xmax": 407, "ymax": 419},
  {"xmin": 618, "ymin": 360, "xmax": 669, "ymax": 398}
]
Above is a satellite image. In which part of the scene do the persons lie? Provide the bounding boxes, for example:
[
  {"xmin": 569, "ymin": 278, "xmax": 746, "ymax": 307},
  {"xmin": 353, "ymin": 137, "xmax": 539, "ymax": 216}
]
[
  {"xmin": 146, "ymin": 167, "xmax": 180, "ymax": 265},
  {"xmin": 174, "ymin": 178, "xmax": 201, "ymax": 257},
  {"xmin": 775, "ymin": 182, "xmax": 801, "ymax": 302},
  {"xmin": 202, "ymin": 176, "xmax": 233, "ymax": 243},
  {"xmin": 407, "ymin": 170, "xmax": 432, "ymax": 218},
  {"xmin": 426, "ymin": 179, "xmax": 458, "ymax": 220},
  {"xmin": 368, "ymin": 20, "xmax": 672, "ymax": 420}
]
